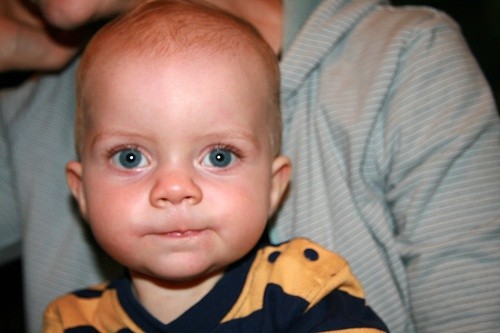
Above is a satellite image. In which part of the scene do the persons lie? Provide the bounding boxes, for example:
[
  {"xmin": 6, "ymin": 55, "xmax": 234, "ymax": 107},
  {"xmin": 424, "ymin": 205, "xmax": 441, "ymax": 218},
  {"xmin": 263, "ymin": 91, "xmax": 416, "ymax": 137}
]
[
  {"xmin": 39, "ymin": 0, "xmax": 392, "ymax": 333},
  {"xmin": 1, "ymin": 0, "xmax": 500, "ymax": 331}
]
